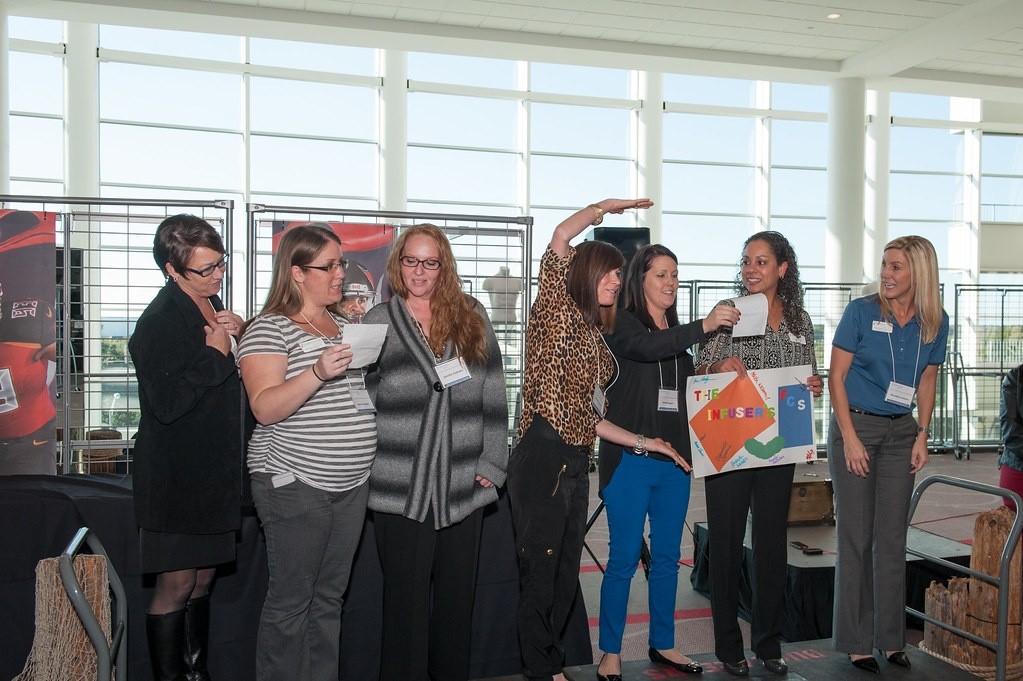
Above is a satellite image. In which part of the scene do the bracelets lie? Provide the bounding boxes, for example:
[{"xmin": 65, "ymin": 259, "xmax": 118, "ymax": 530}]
[
  {"xmin": 312, "ymin": 364, "xmax": 325, "ymax": 381},
  {"xmin": 916, "ymin": 426, "xmax": 928, "ymax": 434},
  {"xmin": 643, "ymin": 437, "xmax": 647, "ymax": 458},
  {"xmin": 709, "ymin": 362, "xmax": 716, "ymax": 373},
  {"xmin": 706, "ymin": 363, "xmax": 712, "ymax": 374}
]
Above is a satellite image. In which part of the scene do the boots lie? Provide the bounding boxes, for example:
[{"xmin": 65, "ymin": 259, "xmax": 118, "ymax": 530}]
[{"xmin": 145, "ymin": 593, "xmax": 211, "ymax": 681}]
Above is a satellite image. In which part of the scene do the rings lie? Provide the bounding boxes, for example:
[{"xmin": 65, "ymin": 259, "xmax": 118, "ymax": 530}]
[
  {"xmin": 820, "ymin": 388, "xmax": 823, "ymax": 394},
  {"xmin": 234, "ymin": 324, "xmax": 237, "ymax": 330}
]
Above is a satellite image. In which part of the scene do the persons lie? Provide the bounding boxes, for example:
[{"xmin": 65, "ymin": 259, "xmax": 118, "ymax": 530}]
[
  {"xmin": 236, "ymin": 225, "xmax": 378, "ymax": 681},
  {"xmin": 506, "ymin": 197, "xmax": 653, "ymax": 681},
  {"xmin": 127, "ymin": 215, "xmax": 247, "ymax": 681},
  {"xmin": 359, "ymin": 224, "xmax": 507, "ymax": 681},
  {"xmin": 1000, "ymin": 365, "xmax": 1023, "ymax": 511},
  {"xmin": 597, "ymin": 244, "xmax": 741, "ymax": 681},
  {"xmin": 827, "ymin": 236, "xmax": 949, "ymax": 675},
  {"xmin": 692, "ymin": 230, "xmax": 823, "ymax": 678}
]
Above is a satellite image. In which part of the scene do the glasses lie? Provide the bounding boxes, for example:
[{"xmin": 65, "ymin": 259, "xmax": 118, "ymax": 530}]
[
  {"xmin": 300, "ymin": 257, "xmax": 349, "ymax": 275},
  {"xmin": 185, "ymin": 252, "xmax": 229, "ymax": 278},
  {"xmin": 399, "ymin": 255, "xmax": 442, "ymax": 270}
]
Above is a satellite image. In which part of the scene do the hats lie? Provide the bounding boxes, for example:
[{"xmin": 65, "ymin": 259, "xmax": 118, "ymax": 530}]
[{"xmin": 342, "ymin": 283, "xmax": 374, "ymax": 298}]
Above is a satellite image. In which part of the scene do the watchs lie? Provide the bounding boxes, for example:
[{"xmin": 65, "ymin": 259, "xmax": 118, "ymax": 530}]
[
  {"xmin": 633, "ymin": 434, "xmax": 643, "ymax": 455},
  {"xmin": 587, "ymin": 204, "xmax": 602, "ymax": 226}
]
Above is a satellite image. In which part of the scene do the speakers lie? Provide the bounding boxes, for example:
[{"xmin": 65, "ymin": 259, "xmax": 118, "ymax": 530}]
[{"xmin": 584, "ymin": 227, "xmax": 651, "ymax": 309}]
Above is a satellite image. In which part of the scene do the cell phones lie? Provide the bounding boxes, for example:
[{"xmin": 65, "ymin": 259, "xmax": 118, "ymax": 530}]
[{"xmin": 790, "ymin": 541, "xmax": 807, "ymax": 548}]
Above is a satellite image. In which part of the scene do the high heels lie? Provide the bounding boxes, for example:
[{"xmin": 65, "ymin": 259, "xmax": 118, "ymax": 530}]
[
  {"xmin": 848, "ymin": 653, "xmax": 881, "ymax": 674},
  {"xmin": 879, "ymin": 649, "xmax": 912, "ymax": 669}
]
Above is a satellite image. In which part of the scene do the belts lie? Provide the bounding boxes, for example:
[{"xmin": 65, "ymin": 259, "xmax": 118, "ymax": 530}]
[{"xmin": 850, "ymin": 409, "xmax": 907, "ymax": 420}]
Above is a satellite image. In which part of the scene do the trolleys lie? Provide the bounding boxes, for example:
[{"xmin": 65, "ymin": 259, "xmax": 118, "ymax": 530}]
[
  {"xmin": 948, "ymin": 352, "xmax": 1007, "ymax": 460},
  {"xmin": 562, "ymin": 474, "xmax": 1023, "ymax": 681}
]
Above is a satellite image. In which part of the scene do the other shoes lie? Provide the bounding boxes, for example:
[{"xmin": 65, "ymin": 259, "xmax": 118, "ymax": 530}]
[
  {"xmin": 648, "ymin": 647, "xmax": 703, "ymax": 676},
  {"xmin": 596, "ymin": 653, "xmax": 622, "ymax": 681},
  {"xmin": 724, "ymin": 660, "xmax": 750, "ymax": 676},
  {"xmin": 758, "ymin": 658, "xmax": 789, "ymax": 672}
]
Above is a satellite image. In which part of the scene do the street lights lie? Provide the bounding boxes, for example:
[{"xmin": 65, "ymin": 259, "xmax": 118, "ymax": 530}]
[{"xmin": 109, "ymin": 393, "xmax": 121, "ymax": 430}]
